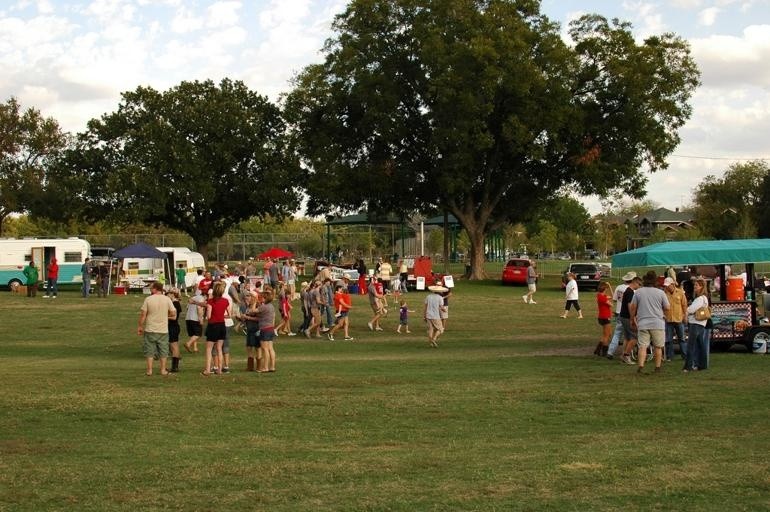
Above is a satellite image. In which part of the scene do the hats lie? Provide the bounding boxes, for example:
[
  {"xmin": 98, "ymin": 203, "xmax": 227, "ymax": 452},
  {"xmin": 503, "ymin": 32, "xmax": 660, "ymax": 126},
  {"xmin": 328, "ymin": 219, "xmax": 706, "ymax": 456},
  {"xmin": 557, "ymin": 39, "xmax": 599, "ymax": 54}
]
[
  {"xmin": 165, "ymin": 287, "xmax": 179, "ymax": 294},
  {"xmin": 343, "ymin": 273, "xmax": 351, "ymax": 280},
  {"xmin": 622, "ymin": 272, "xmax": 636, "ymax": 281},
  {"xmin": 643, "ymin": 271, "xmax": 656, "ymax": 283},
  {"xmin": 663, "ymin": 277, "xmax": 675, "ymax": 286},
  {"xmin": 428, "ymin": 285, "xmax": 449, "ymax": 293}
]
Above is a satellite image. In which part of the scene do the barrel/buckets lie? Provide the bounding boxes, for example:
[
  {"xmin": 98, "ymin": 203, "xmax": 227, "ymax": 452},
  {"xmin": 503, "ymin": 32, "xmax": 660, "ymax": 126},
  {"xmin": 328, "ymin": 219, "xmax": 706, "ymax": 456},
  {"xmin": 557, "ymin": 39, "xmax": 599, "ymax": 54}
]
[
  {"xmin": 726, "ymin": 277, "xmax": 744, "ymax": 301},
  {"xmin": 89, "ymin": 288, "xmax": 95, "ymax": 294},
  {"xmin": 113, "ymin": 286, "xmax": 125, "ymax": 294}
]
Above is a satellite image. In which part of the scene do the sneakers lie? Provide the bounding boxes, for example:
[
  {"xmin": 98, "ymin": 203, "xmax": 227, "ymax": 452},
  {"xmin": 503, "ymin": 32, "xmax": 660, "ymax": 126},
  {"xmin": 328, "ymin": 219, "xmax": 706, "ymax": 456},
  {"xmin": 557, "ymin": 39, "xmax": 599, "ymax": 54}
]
[
  {"xmin": 335, "ymin": 312, "xmax": 341, "ymax": 317},
  {"xmin": 396, "ymin": 330, "xmax": 410, "ymax": 334},
  {"xmin": 274, "ymin": 324, "xmax": 354, "ymax": 341},
  {"xmin": 385, "ymin": 289, "xmax": 408, "ymax": 296},
  {"xmin": 594, "ymin": 342, "xmax": 699, "ymax": 375},
  {"xmin": 247, "ymin": 357, "xmax": 275, "ymax": 376},
  {"xmin": 42, "ymin": 295, "xmax": 57, "ymax": 299},
  {"xmin": 368, "ymin": 304, "xmax": 388, "ymax": 331},
  {"xmin": 234, "ymin": 321, "xmax": 247, "ymax": 336},
  {"xmin": 200, "ymin": 366, "xmax": 230, "ymax": 376},
  {"xmin": 393, "ymin": 300, "xmax": 398, "ymax": 303},
  {"xmin": 523, "ymin": 295, "xmax": 536, "ymax": 304},
  {"xmin": 426, "ymin": 336, "xmax": 440, "ymax": 347},
  {"xmin": 560, "ymin": 315, "xmax": 583, "ymax": 320}
]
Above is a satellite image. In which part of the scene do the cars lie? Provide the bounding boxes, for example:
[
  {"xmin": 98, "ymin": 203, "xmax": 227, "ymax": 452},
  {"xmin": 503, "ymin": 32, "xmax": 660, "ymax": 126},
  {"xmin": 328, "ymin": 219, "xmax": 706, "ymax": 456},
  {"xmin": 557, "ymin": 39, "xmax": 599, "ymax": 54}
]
[
  {"xmin": 560, "ymin": 263, "xmax": 601, "ymax": 291},
  {"xmin": 502, "ymin": 259, "xmax": 538, "ymax": 286}
]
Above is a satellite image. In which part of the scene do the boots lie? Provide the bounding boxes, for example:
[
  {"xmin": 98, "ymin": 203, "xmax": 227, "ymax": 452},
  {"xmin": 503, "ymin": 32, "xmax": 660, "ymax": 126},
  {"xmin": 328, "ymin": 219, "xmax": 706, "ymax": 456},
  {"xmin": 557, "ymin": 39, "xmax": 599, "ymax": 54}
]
[{"xmin": 146, "ymin": 356, "xmax": 180, "ymax": 375}]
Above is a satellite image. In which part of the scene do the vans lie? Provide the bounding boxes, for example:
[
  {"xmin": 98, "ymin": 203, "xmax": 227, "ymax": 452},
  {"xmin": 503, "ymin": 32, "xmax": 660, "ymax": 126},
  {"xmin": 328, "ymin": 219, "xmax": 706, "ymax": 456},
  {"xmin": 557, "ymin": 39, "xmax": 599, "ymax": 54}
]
[{"xmin": 87, "ymin": 246, "xmax": 118, "ymax": 275}]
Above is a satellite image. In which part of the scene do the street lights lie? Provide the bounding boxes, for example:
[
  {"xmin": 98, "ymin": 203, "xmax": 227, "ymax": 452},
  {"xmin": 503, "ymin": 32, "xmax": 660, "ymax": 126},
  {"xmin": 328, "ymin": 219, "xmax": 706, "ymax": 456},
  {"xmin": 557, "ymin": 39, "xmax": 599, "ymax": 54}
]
[{"xmin": 325, "ymin": 214, "xmax": 335, "ymax": 263}]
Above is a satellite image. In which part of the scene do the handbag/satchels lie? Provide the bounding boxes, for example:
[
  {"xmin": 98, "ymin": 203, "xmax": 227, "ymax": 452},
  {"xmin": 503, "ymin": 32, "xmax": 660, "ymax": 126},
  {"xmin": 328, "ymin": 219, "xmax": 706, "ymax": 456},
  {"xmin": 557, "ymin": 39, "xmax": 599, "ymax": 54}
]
[{"xmin": 695, "ymin": 307, "xmax": 712, "ymax": 320}]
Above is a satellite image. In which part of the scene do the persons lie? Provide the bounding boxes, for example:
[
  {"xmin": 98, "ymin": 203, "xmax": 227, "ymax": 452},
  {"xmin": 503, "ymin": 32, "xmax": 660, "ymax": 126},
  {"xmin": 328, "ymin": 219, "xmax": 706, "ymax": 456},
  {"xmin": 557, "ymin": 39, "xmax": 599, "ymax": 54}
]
[
  {"xmin": 396, "ymin": 299, "xmax": 415, "ymax": 334},
  {"xmin": 520, "ymin": 260, "xmax": 540, "ymax": 304},
  {"xmin": 136, "ymin": 255, "xmax": 392, "ymax": 377},
  {"xmin": 435, "ymin": 281, "xmax": 451, "ymax": 331},
  {"xmin": 422, "ymin": 286, "xmax": 447, "ymax": 347},
  {"xmin": 80, "ymin": 257, "xmax": 93, "ymax": 298},
  {"xmin": 397, "ymin": 259, "xmax": 409, "ymax": 293},
  {"xmin": 41, "ymin": 256, "xmax": 58, "ymax": 299},
  {"xmin": 93, "ymin": 263, "xmax": 109, "ymax": 298},
  {"xmin": 593, "ymin": 264, "xmax": 770, "ymax": 373},
  {"xmin": 23, "ymin": 261, "xmax": 39, "ymax": 297},
  {"xmin": 560, "ymin": 272, "xmax": 583, "ymax": 319}
]
